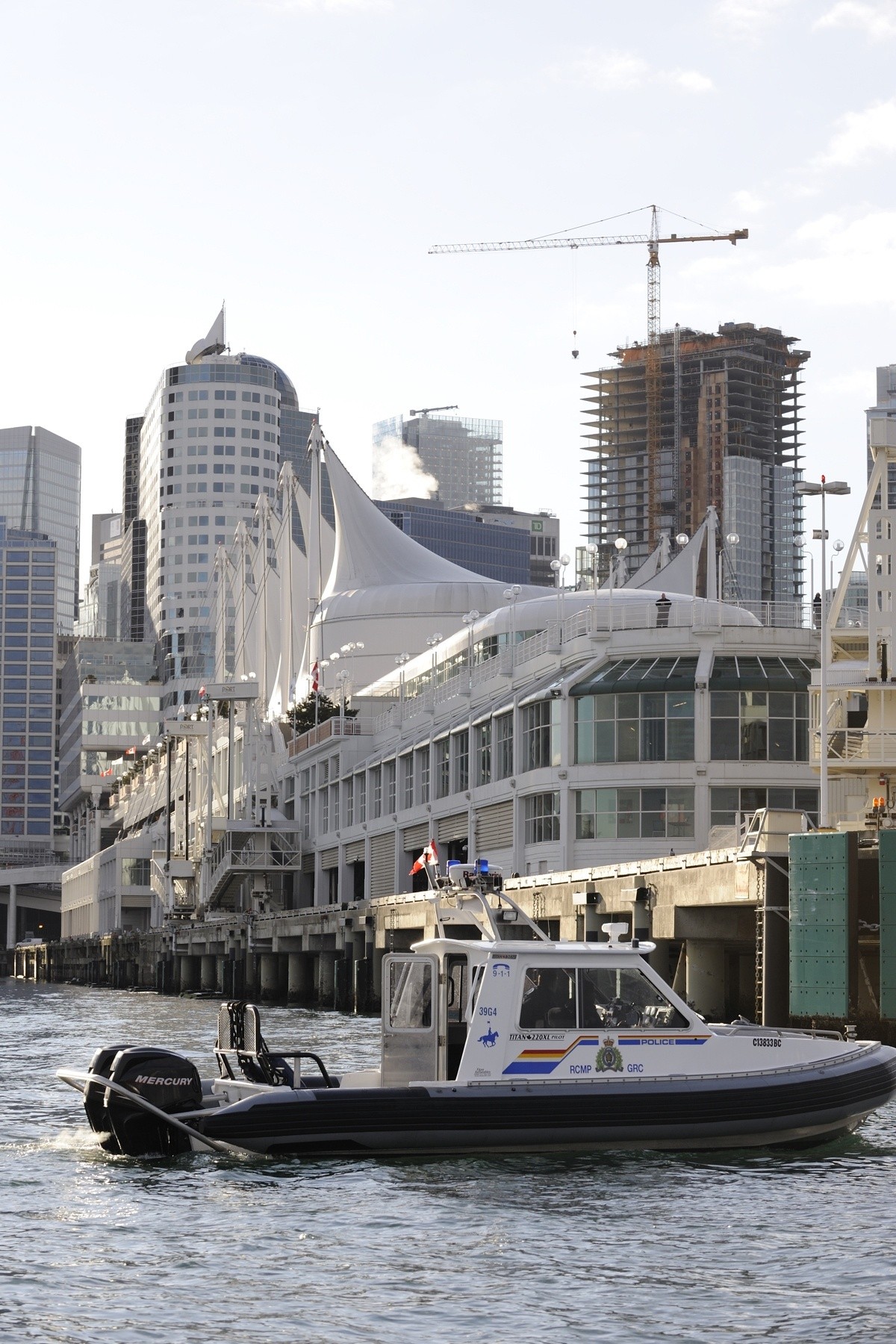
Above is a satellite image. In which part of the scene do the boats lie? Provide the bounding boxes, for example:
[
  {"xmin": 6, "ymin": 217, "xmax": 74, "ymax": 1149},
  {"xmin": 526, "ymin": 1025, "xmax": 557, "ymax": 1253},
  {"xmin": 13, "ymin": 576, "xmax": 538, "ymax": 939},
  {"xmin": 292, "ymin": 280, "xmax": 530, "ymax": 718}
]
[{"xmin": 52, "ymin": 846, "xmax": 896, "ymax": 1164}]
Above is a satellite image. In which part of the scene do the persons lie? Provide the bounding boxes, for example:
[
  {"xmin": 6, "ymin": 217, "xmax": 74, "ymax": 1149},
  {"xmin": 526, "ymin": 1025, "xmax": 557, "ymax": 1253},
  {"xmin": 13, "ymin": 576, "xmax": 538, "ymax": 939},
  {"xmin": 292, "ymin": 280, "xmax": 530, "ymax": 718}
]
[
  {"xmin": 813, "ymin": 593, "xmax": 821, "ymax": 629},
  {"xmin": 655, "ymin": 593, "xmax": 672, "ymax": 627},
  {"xmin": 523, "ymin": 969, "xmax": 604, "ymax": 1028}
]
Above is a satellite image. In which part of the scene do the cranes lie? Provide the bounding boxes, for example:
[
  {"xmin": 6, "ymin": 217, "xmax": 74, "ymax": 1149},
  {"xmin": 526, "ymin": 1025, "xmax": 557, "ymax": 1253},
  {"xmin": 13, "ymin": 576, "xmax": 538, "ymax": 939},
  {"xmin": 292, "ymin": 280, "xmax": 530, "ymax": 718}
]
[
  {"xmin": 423, "ymin": 202, "xmax": 752, "ymax": 558},
  {"xmin": 408, "ymin": 405, "xmax": 460, "ymax": 417}
]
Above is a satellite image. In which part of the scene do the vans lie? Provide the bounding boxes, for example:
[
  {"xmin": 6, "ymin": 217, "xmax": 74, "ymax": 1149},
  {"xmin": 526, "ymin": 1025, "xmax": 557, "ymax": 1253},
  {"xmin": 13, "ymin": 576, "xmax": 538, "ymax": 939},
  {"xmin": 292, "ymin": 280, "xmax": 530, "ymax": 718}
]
[{"xmin": 16, "ymin": 937, "xmax": 49, "ymax": 948}]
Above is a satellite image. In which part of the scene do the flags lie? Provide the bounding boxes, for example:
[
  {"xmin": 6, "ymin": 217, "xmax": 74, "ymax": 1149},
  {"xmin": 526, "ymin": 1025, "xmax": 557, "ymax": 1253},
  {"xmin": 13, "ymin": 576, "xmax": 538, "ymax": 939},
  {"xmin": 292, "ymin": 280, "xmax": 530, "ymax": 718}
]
[
  {"xmin": 101, "ymin": 768, "xmax": 112, "ymax": 777},
  {"xmin": 311, "ymin": 662, "xmax": 319, "ymax": 693},
  {"xmin": 199, "ymin": 687, "xmax": 205, "ymax": 696},
  {"xmin": 126, "ymin": 746, "xmax": 136, "ymax": 754},
  {"xmin": 408, "ymin": 840, "xmax": 438, "ymax": 876},
  {"xmin": 142, "ymin": 734, "xmax": 150, "ymax": 746},
  {"xmin": 111, "ymin": 757, "xmax": 123, "ymax": 765}
]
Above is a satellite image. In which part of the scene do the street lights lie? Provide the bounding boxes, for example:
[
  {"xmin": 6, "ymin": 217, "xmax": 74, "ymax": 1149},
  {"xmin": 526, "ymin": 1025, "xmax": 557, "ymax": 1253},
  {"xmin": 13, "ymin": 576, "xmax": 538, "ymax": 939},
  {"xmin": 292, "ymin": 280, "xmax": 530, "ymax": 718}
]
[
  {"xmin": 559, "ymin": 554, "xmax": 571, "ymax": 646},
  {"xmin": 503, "ymin": 585, "xmax": 522, "ymax": 648},
  {"xmin": 462, "ymin": 609, "xmax": 479, "ymax": 676},
  {"xmin": 117, "ymin": 776, "xmax": 123, "ymax": 794},
  {"xmin": 586, "ymin": 543, "xmax": 598, "ymax": 633},
  {"xmin": 155, "ymin": 742, "xmax": 163, "ymax": 764},
  {"xmin": 718, "ymin": 532, "xmax": 741, "ymax": 603},
  {"xmin": 794, "ymin": 474, "xmax": 853, "ymax": 827},
  {"xmin": 395, "ymin": 652, "xmax": 410, "ymax": 704},
  {"xmin": 549, "ymin": 560, "xmax": 562, "ymax": 646},
  {"xmin": 314, "ymin": 686, "xmax": 325, "ymax": 745},
  {"xmin": 336, "ymin": 670, "xmax": 350, "ymax": 735},
  {"xmin": 141, "ymin": 755, "xmax": 148, "ymax": 776},
  {"xmin": 607, "ymin": 537, "xmax": 628, "ymax": 631}
]
